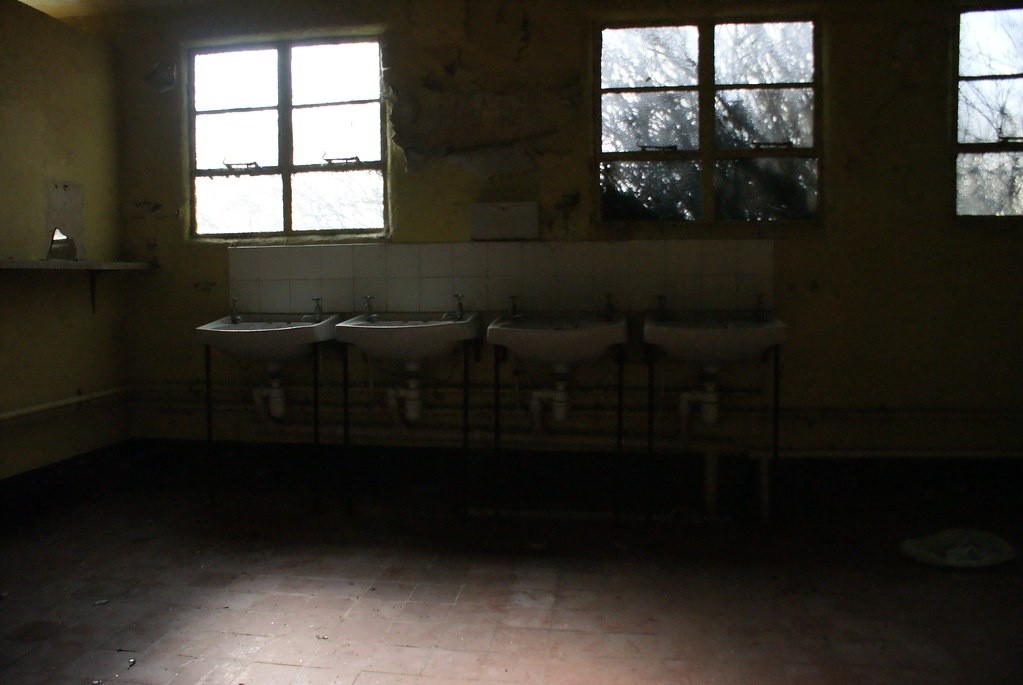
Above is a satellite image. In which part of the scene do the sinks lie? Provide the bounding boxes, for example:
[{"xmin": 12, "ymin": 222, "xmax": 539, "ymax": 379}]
[
  {"xmin": 334, "ymin": 313, "xmax": 479, "ymax": 362},
  {"xmin": 644, "ymin": 318, "xmax": 786, "ymax": 364},
  {"xmin": 487, "ymin": 314, "xmax": 627, "ymax": 363},
  {"xmin": 197, "ymin": 315, "xmax": 337, "ymax": 364}
]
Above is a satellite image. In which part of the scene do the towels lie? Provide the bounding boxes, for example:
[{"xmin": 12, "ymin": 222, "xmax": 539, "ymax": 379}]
[{"xmin": 899, "ymin": 525, "xmax": 1013, "ymax": 571}]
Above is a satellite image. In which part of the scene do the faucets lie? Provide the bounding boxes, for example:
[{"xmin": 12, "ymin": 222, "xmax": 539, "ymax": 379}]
[
  {"xmin": 649, "ymin": 292, "xmax": 676, "ymax": 322},
  {"xmin": 300, "ymin": 296, "xmax": 323, "ymax": 322},
  {"xmin": 359, "ymin": 295, "xmax": 380, "ymax": 324},
  {"xmin": 594, "ymin": 292, "xmax": 617, "ymax": 322},
  {"xmin": 441, "ymin": 294, "xmax": 465, "ymax": 322},
  {"xmin": 745, "ymin": 292, "xmax": 770, "ymax": 322},
  {"xmin": 227, "ymin": 297, "xmax": 243, "ymax": 324},
  {"xmin": 505, "ymin": 295, "xmax": 531, "ymax": 321}
]
